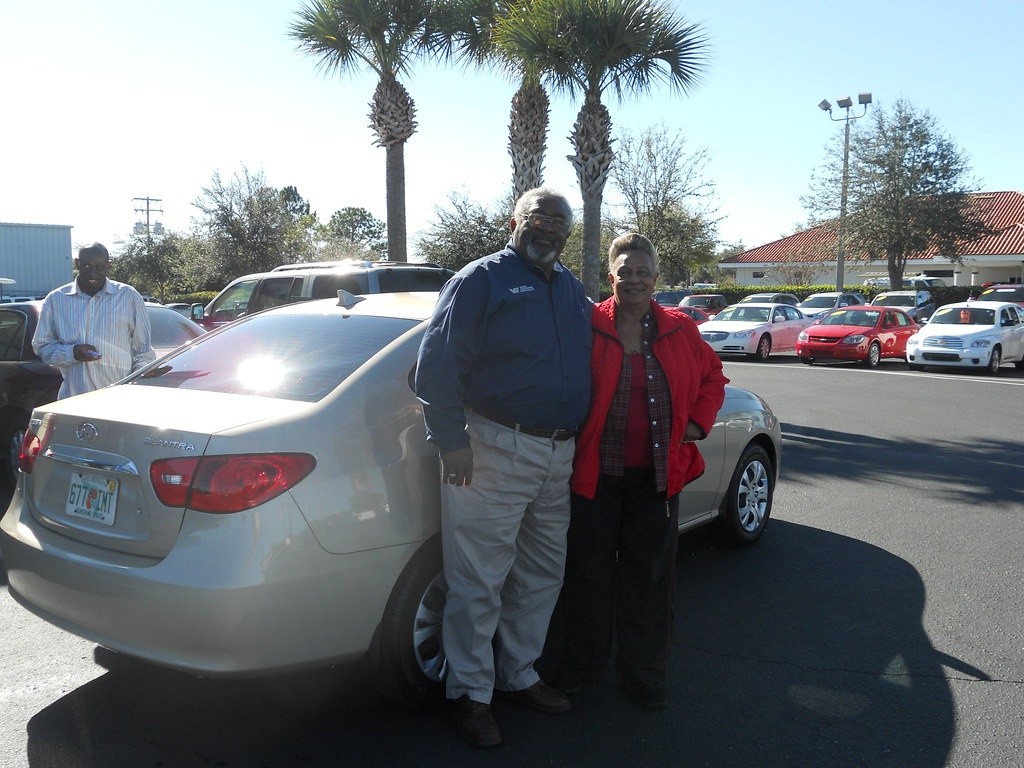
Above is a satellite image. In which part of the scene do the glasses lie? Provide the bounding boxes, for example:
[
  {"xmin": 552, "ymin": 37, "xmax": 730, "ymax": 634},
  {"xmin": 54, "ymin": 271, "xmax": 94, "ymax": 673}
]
[{"xmin": 518, "ymin": 213, "xmax": 569, "ymax": 231}]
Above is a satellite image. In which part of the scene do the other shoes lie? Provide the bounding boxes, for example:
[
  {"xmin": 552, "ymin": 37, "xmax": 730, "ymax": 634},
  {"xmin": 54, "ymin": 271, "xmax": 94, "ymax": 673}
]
[{"xmin": 620, "ymin": 662, "xmax": 667, "ymax": 708}]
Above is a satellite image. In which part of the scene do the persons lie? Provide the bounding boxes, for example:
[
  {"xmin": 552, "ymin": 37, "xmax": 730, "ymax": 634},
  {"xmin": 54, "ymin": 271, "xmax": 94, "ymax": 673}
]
[
  {"xmin": 413, "ymin": 188, "xmax": 595, "ymax": 745},
  {"xmin": 560, "ymin": 233, "xmax": 730, "ymax": 707},
  {"xmin": 31, "ymin": 242, "xmax": 156, "ymax": 401}
]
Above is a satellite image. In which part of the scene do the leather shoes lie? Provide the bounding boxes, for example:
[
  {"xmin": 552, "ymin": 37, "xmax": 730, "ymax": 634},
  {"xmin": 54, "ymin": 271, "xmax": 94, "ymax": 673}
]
[
  {"xmin": 449, "ymin": 699, "xmax": 502, "ymax": 747},
  {"xmin": 492, "ymin": 679, "xmax": 571, "ymax": 713}
]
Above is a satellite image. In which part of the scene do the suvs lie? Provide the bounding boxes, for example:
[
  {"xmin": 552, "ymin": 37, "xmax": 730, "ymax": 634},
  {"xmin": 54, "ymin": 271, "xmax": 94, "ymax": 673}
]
[
  {"xmin": 650, "ymin": 288, "xmax": 693, "ymax": 307},
  {"xmin": 864, "ymin": 290, "xmax": 937, "ymax": 325},
  {"xmin": 0, "ymin": 254, "xmax": 459, "ymax": 509}
]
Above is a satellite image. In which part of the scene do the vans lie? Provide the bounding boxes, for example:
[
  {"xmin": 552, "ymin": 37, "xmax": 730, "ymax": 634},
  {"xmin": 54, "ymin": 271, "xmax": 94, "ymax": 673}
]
[
  {"xmin": 677, "ymin": 294, "xmax": 728, "ymax": 316},
  {"xmin": 863, "ymin": 273, "xmax": 947, "ymax": 289},
  {"xmin": 971, "ymin": 283, "xmax": 1024, "ymax": 319}
]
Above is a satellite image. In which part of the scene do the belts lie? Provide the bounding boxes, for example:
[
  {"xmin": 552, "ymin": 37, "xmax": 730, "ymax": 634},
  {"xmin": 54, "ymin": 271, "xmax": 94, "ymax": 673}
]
[{"xmin": 470, "ymin": 404, "xmax": 578, "ymax": 440}]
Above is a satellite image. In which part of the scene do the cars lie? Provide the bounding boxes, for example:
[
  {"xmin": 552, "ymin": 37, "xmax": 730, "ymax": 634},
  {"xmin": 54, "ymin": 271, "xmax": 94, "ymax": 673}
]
[
  {"xmin": 906, "ymin": 300, "xmax": 1024, "ymax": 377},
  {"xmin": 696, "ymin": 302, "xmax": 815, "ymax": 363},
  {"xmin": 735, "ymin": 292, "xmax": 800, "ymax": 320},
  {"xmin": 792, "ymin": 291, "xmax": 866, "ymax": 319},
  {"xmin": 796, "ymin": 304, "xmax": 923, "ymax": 370},
  {"xmin": 0, "ymin": 289, "xmax": 783, "ymax": 716},
  {"xmin": 672, "ymin": 304, "xmax": 710, "ymax": 326}
]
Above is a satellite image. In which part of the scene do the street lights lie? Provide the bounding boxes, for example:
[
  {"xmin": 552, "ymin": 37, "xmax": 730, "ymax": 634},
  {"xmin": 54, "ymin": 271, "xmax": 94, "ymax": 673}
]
[{"xmin": 818, "ymin": 91, "xmax": 873, "ymax": 294}]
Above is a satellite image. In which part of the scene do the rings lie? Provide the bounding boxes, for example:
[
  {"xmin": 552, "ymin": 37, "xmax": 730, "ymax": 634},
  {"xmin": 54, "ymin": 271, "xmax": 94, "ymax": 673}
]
[
  {"xmin": 682, "ymin": 440, "xmax": 693, "ymax": 444},
  {"xmin": 449, "ymin": 474, "xmax": 456, "ymax": 476}
]
